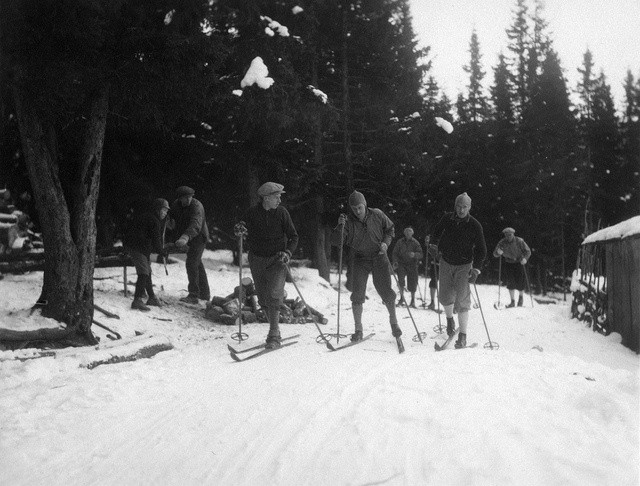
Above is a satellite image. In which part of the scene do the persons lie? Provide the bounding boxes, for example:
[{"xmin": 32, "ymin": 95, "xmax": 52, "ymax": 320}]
[
  {"xmin": 164, "ymin": 185, "xmax": 212, "ymax": 309},
  {"xmin": 428, "ymin": 251, "xmax": 443, "ymax": 314},
  {"xmin": 6, "ymin": 215, "xmax": 34, "ymax": 275},
  {"xmin": 429, "ymin": 191, "xmax": 487, "ymax": 348},
  {"xmin": 328, "ymin": 190, "xmax": 403, "ymax": 342},
  {"xmin": 493, "ymin": 227, "xmax": 532, "ymax": 308},
  {"xmin": 122, "ymin": 198, "xmax": 170, "ymax": 311},
  {"xmin": 392, "ymin": 225, "xmax": 424, "ymax": 309},
  {"xmin": 233, "ymin": 182, "xmax": 299, "ymax": 350}
]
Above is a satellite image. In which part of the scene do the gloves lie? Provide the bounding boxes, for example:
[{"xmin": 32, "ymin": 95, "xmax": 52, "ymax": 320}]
[
  {"xmin": 161, "ymin": 248, "xmax": 168, "ymax": 257},
  {"xmin": 233, "ymin": 221, "xmax": 248, "ymax": 240},
  {"xmin": 469, "ymin": 268, "xmax": 481, "ymax": 283},
  {"xmin": 429, "ymin": 244, "xmax": 438, "ymax": 258},
  {"xmin": 279, "ymin": 250, "xmax": 292, "ymax": 265},
  {"xmin": 497, "ymin": 248, "xmax": 503, "ymax": 256},
  {"xmin": 175, "ymin": 234, "xmax": 189, "ymax": 247},
  {"xmin": 520, "ymin": 258, "xmax": 527, "ymax": 265}
]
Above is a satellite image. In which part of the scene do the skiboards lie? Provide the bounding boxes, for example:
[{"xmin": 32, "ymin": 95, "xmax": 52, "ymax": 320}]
[
  {"xmin": 428, "ymin": 304, "xmax": 457, "ymax": 314},
  {"xmin": 395, "ymin": 302, "xmax": 416, "ymax": 309},
  {"xmin": 434, "ymin": 326, "xmax": 477, "ymax": 351},
  {"xmin": 494, "ymin": 302, "xmax": 514, "ymax": 313},
  {"xmin": 226, "ymin": 334, "xmax": 301, "ymax": 363},
  {"xmin": 327, "ymin": 330, "xmax": 407, "ymax": 354}
]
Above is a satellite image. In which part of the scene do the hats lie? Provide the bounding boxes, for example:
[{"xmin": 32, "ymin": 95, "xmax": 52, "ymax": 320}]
[
  {"xmin": 177, "ymin": 186, "xmax": 195, "ymax": 196},
  {"xmin": 154, "ymin": 198, "xmax": 171, "ymax": 211},
  {"xmin": 258, "ymin": 182, "xmax": 286, "ymax": 196},
  {"xmin": 455, "ymin": 192, "xmax": 471, "ymax": 210},
  {"xmin": 503, "ymin": 227, "xmax": 515, "ymax": 233},
  {"xmin": 349, "ymin": 190, "xmax": 366, "ymax": 207}
]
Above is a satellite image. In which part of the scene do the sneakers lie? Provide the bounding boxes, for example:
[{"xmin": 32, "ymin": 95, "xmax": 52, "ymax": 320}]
[
  {"xmin": 399, "ymin": 298, "xmax": 403, "ymax": 303},
  {"xmin": 147, "ymin": 298, "xmax": 160, "ymax": 306},
  {"xmin": 132, "ymin": 298, "xmax": 150, "ymax": 311},
  {"xmin": 391, "ymin": 324, "xmax": 402, "ymax": 337},
  {"xmin": 455, "ymin": 333, "xmax": 466, "ymax": 348},
  {"xmin": 266, "ymin": 330, "xmax": 281, "ymax": 348},
  {"xmin": 518, "ymin": 300, "xmax": 522, "ymax": 306},
  {"xmin": 427, "ymin": 304, "xmax": 435, "ymax": 308},
  {"xmin": 447, "ymin": 317, "xmax": 455, "ymax": 336},
  {"xmin": 351, "ymin": 330, "xmax": 363, "ymax": 341},
  {"xmin": 509, "ymin": 302, "xmax": 515, "ymax": 307},
  {"xmin": 410, "ymin": 303, "xmax": 417, "ymax": 309},
  {"xmin": 199, "ymin": 295, "xmax": 210, "ymax": 301},
  {"xmin": 179, "ymin": 294, "xmax": 198, "ymax": 304}
]
